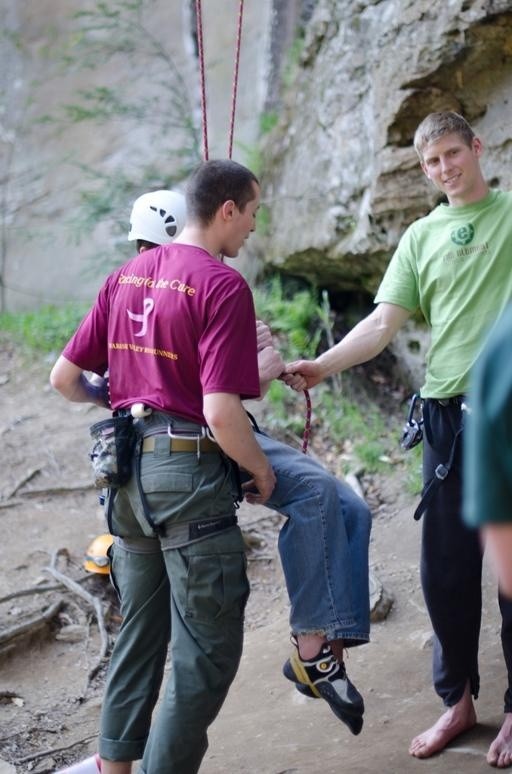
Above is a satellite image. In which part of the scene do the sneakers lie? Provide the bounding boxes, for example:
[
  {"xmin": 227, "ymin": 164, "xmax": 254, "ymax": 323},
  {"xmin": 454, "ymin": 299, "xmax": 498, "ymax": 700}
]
[
  {"xmin": 284, "ymin": 640, "xmax": 363, "ymax": 711},
  {"xmin": 296, "ymin": 681, "xmax": 364, "ymax": 735}
]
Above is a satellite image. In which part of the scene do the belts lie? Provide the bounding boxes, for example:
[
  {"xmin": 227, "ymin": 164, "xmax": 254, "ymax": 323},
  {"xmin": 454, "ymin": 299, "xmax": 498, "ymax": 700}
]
[{"xmin": 138, "ymin": 438, "xmax": 221, "ymax": 454}]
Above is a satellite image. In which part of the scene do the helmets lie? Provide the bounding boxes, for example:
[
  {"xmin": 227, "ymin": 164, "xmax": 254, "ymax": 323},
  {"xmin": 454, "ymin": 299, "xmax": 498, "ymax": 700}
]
[
  {"xmin": 130, "ymin": 191, "xmax": 189, "ymax": 246},
  {"xmin": 82, "ymin": 533, "xmax": 115, "ymax": 575}
]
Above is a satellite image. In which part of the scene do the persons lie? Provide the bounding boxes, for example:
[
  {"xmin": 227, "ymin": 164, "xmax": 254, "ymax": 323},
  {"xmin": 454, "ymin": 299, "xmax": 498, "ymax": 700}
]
[
  {"xmin": 460, "ymin": 296, "xmax": 512, "ymax": 599},
  {"xmin": 282, "ymin": 109, "xmax": 512, "ymax": 768},
  {"xmin": 128, "ymin": 190, "xmax": 373, "ymax": 736},
  {"xmin": 48, "ymin": 159, "xmax": 277, "ymax": 774}
]
